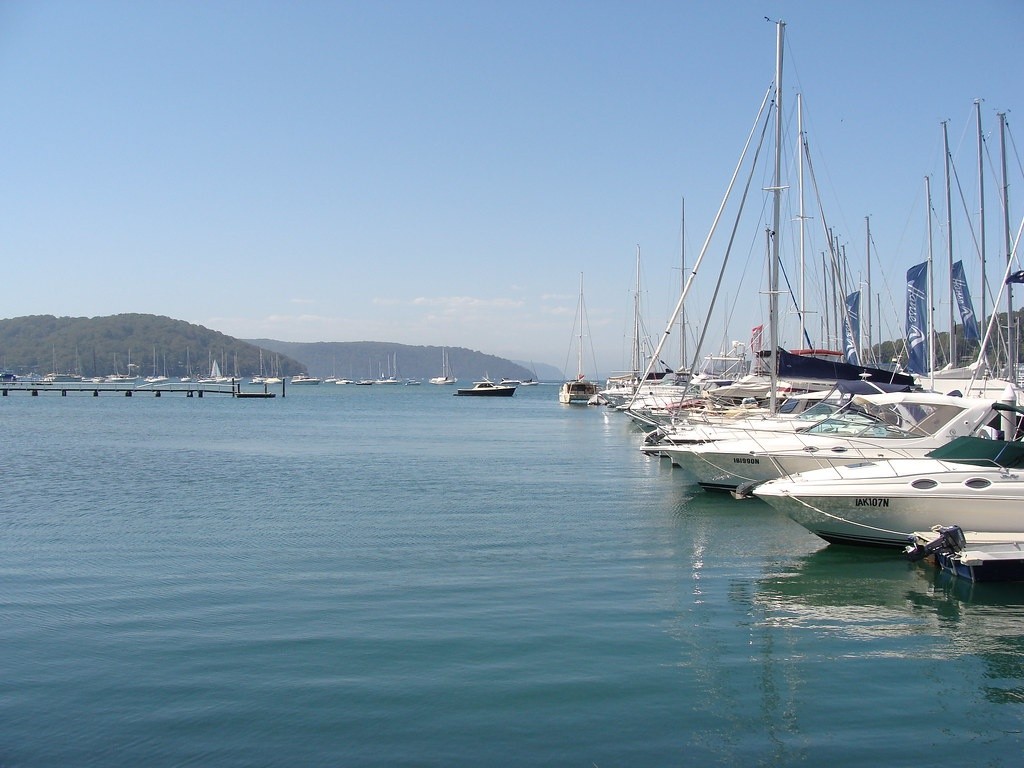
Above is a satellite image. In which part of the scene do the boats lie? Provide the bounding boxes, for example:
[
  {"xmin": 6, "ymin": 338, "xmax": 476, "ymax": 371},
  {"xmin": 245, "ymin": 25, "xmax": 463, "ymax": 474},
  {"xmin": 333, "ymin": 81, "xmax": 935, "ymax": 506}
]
[
  {"xmin": 473, "ymin": 371, "xmax": 496, "ymax": 384},
  {"xmin": 452, "ymin": 380, "xmax": 517, "ymax": 397},
  {"xmin": 290, "ymin": 374, "xmax": 321, "ymax": 386},
  {"xmin": 520, "ymin": 381, "xmax": 540, "ymax": 386},
  {"xmin": 500, "ymin": 378, "xmax": 520, "ymax": 385}
]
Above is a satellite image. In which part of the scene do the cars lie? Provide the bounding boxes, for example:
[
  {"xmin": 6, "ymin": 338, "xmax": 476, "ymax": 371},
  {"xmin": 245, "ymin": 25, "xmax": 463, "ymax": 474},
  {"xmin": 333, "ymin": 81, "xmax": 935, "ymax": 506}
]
[{"xmin": 0, "ymin": 374, "xmax": 17, "ymax": 385}]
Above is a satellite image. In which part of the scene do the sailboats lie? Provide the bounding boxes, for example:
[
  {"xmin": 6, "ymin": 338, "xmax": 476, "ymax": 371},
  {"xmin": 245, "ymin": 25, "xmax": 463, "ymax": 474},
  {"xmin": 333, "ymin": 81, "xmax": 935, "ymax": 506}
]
[
  {"xmin": 323, "ymin": 350, "xmax": 422, "ymax": 386},
  {"xmin": 43, "ymin": 343, "xmax": 282, "ymax": 384},
  {"xmin": 429, "ymin": 346, "xmax": 457, "ymax": 385},
  {"xmin": 558, "ymin": 14, "xmax": 1024, "ymax": 553}
]
